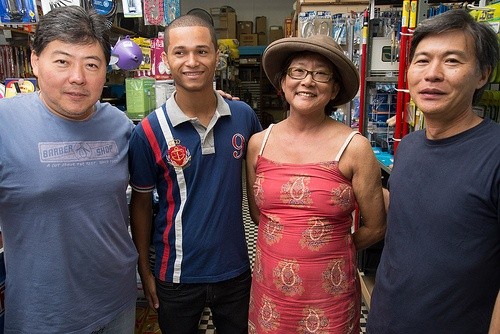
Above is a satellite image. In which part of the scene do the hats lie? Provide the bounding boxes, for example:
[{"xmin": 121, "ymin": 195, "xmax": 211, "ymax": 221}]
[{"xmin": 262, "ymin": 36, "xmax": 359, "ymax": 106}]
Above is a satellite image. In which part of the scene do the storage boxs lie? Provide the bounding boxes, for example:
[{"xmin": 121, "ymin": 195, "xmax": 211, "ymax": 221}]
[
  {"xmin": 121, "ymin": 76, "xmax": 157, "ymax": 122},
  {"xmin": 257, "ymin": 33, "xmax": 268, "ymax": 45},
  {"xmin": 220, "ymin": 13, "xmax": 236, "ymax": 41},
  {"xmin": 371, "ymin": 36, "xmax": 400, "ymax": 72},
  {"xmin": 237, "ymin": 20, "xmax": 253, "ymax": 40},
  {"xmin": 239, "ymin": 35, "xmax": 257, "ymax": 46},
  {"xmin": 268, "ymin": 29, "xmax": 284, "ymax": 44},
  {"xmin": 284, "ymin": 17, "xmax": 291, "ymax": 38},
  {"xmin": 255, "ymin": 16, "xmax": 267, "ymax": 33}
]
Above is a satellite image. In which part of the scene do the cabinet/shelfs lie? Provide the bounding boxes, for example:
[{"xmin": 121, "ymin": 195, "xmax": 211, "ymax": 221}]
[{"xmin": 0, "ymin": 0, "xmax": 500, "ymax": 306}]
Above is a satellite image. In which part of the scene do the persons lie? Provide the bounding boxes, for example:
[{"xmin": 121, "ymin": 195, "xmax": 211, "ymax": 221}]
[
  {"xmin": 129, "ymin": 16, "xmax": 265, "ymax": 334},
  {"xmin": 0, "ymin": 6, "xmax": 240, "ymax": 334},
  {"xmin": 245, "ymin": 36, "xmax": 387, "ymax": 334},
  {"xmin": 383, "ymin": 7, "xmax": 500, "ymax": 334}
]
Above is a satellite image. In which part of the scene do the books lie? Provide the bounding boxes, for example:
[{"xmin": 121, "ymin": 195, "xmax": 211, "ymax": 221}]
[{"xmin": 0, "ymin": 44, "xmax": 34, "ymax": 79}]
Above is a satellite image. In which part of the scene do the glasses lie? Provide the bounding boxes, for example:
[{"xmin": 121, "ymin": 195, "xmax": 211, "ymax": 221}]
[{"xmin": 286, "ymin": 66, "xmax": 334, "ymax": 83}]
